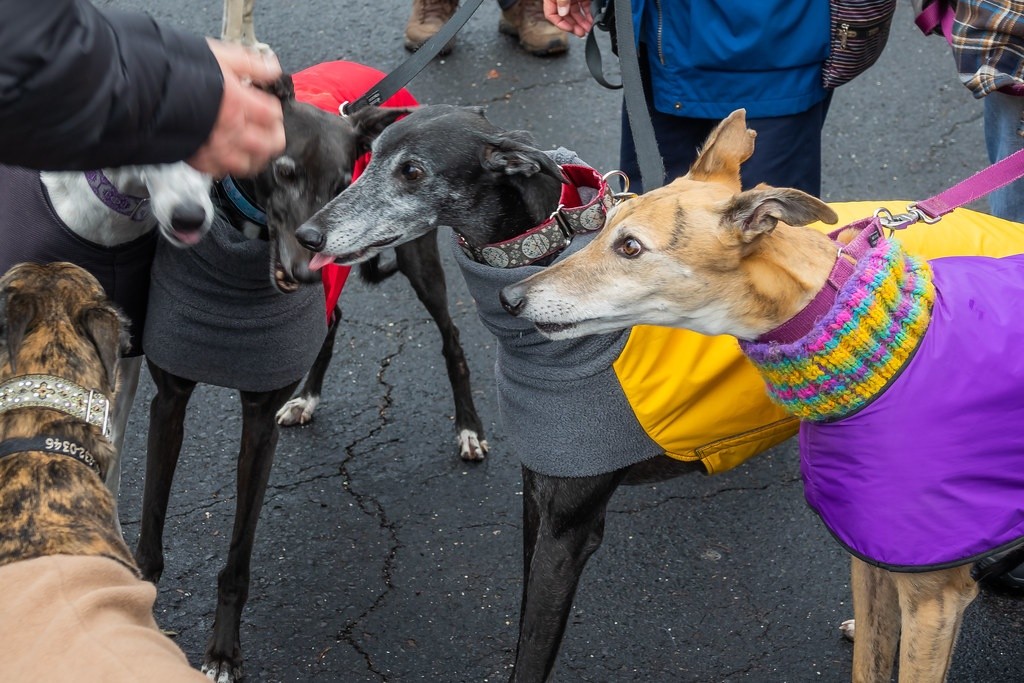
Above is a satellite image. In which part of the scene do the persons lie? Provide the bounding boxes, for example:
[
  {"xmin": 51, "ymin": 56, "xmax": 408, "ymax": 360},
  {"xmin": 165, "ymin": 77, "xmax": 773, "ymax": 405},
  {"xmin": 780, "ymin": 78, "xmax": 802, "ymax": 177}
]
[
  {"xmin": 906, "ymin": 0, "xmax": 1024, "ymax": 602},
  {"xmin": 403, "ymin": 0, "xmax": 571, "ymax": 57},
  {"xmin": 542, "ymin": 0, "xmax": 896, "ymax": 201},
  {"xmin": 0, "ymin": 0, "xmax": 289, "ymax": 180}
]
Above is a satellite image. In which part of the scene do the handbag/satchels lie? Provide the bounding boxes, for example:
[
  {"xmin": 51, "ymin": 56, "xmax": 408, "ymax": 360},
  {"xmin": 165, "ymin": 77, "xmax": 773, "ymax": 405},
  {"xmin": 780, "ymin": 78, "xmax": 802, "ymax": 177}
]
[{"xmin": 822, "ymin": 0, "xmax": 898, "ymax": 90}]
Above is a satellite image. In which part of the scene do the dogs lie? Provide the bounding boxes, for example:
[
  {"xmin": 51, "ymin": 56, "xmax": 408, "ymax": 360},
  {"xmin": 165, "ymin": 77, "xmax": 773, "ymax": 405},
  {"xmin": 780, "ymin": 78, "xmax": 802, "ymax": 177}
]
[{"xmin": 0, "ymin": 70, "xmax": 1024, "ymax": 683}]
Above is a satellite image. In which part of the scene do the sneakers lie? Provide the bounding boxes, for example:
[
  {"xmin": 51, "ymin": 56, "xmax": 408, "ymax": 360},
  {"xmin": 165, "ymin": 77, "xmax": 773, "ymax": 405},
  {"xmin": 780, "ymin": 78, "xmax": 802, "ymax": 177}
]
[
  {"xmin": 498, "ymin": 0, "xmax": 570, "ymax": 57},
  {"xmin": 405, "ymin": 0, "xmax": 458, "ymax": 54}
]
